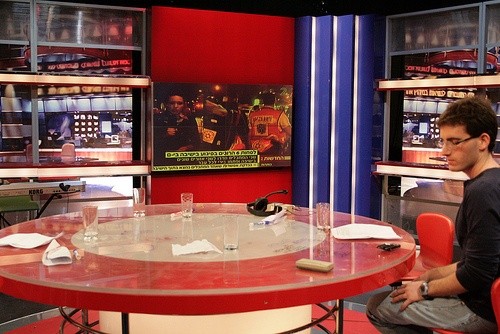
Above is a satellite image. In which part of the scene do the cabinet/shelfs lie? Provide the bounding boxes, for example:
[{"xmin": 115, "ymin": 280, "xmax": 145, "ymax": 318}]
[
  {"xmin": 370, "ymin": 74, "xmax": 500, "ymax": 181},
  {"xmin": 0, "ymin": 70, "xmax": 152, "ymax": 178}
]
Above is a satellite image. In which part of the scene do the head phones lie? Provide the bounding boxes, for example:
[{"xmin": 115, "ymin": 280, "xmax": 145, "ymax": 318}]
[{"xmin": 248, "ymin": 197, "xmax": 284, "ymax": 216}]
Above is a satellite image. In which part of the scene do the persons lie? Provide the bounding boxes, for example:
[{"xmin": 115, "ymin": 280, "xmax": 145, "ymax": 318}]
[
  {"xmin": 153, "ymin": 91, "xmax": 291, "ymax": 157},
  {"xmin": 366, "ymin": 94, "xmax": 500, "ymax": 334}
]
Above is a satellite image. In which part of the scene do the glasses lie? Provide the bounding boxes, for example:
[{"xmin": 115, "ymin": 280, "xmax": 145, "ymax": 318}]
[{"xmin": 433, "ymin": 135, "xmax": 472, "ymax": 149}]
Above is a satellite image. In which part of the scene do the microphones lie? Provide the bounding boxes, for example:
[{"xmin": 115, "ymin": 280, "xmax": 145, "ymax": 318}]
[{"xmin": 264, "ymin": 190, "xmax": 288, "ymax": 199}]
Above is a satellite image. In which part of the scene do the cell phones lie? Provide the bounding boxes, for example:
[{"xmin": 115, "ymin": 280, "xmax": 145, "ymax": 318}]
[{"xmin": 377, "ymin": 243, "xmax": 401, "ymax": 249}]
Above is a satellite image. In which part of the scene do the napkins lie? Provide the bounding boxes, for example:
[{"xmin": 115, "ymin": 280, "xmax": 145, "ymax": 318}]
[
  {"xmin": 41, "ymin": 238, "xmax": 74, "ymax": 266},
  {"xmin": 0, "ymin": 202, "xmax": 419, "ymax": 334},
  {"xmin": 0, "ymin": 228, "xmax": 66, "ymax": 250},
  {"xmin": 330, "ymin": 220, "xmax": 402, "ymax": 242},
  {"xmin": 168, "ymin": 238, "xmax": 225, "ymax": 259}
]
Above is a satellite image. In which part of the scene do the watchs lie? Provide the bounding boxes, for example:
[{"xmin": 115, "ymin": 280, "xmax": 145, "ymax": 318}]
[{"xmin": 420, "ymin": 281, "xmax": 433, "ymax": 301}]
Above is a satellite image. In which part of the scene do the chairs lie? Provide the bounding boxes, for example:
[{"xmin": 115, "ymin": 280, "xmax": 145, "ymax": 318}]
[
  {"xmin": 432, "ymin": 277, "xmax": 500, "ymax": 334},
  {"xmin": 61, "ymin": 143, "xmax": 77, "ymax": 157},
  {"xmin": 399, "ymin": 208, "xmax": 456, "ymax": 284}
]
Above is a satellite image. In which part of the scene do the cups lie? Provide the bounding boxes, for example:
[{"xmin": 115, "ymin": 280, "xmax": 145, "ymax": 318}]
[
  {"xmin": 82, "ymin": 204, "xmax": 99, "ymax": 242},
  {"xmin": 181, "ymin": 192, "xmax": 193, "ymax": 218},
  {"xmin": 316, "ymin": 203, "xmax": 330, "ymax": 232},
  {"xmin": 132, "ymin": 187, "xmax": 145, "ymax": 217},
  {"xmin": 223, "ymin": 215, "xmax": 239, "ymax": 251}
]
